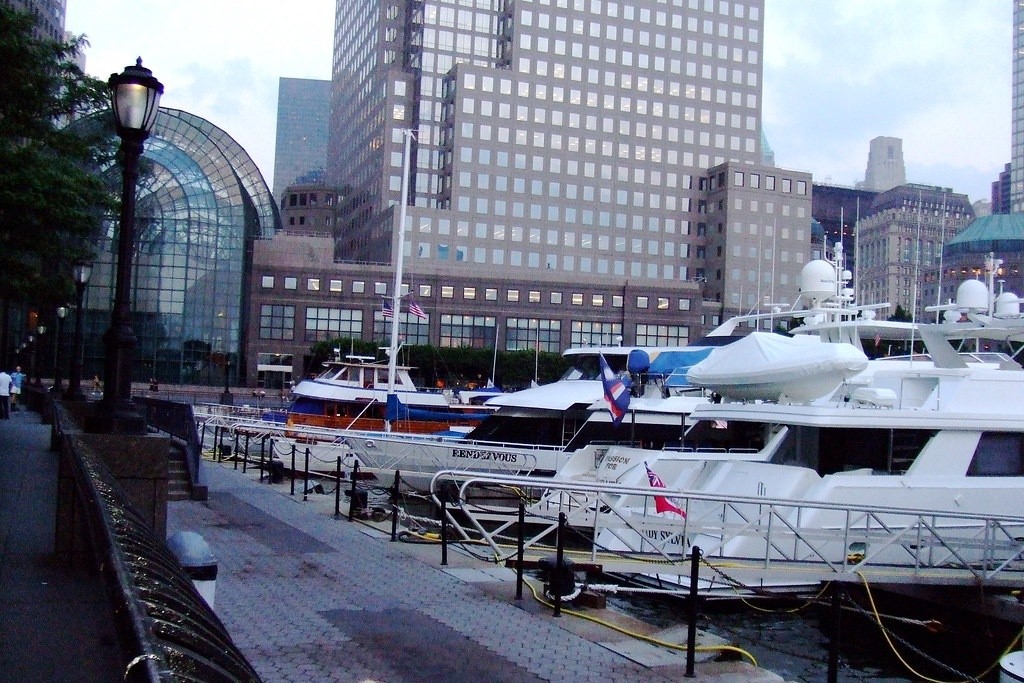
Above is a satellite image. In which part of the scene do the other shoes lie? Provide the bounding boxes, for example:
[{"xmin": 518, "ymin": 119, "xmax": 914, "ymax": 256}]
[
  {"xmin": 0, "ymin": 415, "xmax": 11, "ymax": 419},
  {"xmin": 92, "ymin": 392, "xmax": 95, "ymax": 396},
  {"xmin": 100, "ymin": 392, "xmax": 103, "ymax": 395}
]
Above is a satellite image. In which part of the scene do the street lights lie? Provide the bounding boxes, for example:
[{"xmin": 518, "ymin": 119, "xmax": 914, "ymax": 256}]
[
  {"xmin": 32, "ymin": 320, "xmax": 47, "ymax": 389},
  {"xmin": 49, "ymin": 303, "xmax": 70, "ymax": 393},
  {"xmin": 79, "ymin": 54, "xmax": 165, "ymax": 435},
  {"xmin": 60, "ymin": 260, "xmax": 94, "ymax": 401}
]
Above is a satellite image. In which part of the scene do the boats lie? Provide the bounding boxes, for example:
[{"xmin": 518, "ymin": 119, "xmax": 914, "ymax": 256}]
[{"xmin": 257, "ymin": 198, "xmax": 1024, "ymax": 633}]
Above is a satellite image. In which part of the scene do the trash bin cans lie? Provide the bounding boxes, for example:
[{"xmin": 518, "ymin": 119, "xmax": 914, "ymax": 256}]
[{"xmin": 167, "ymin": 531, "xmax": 219, "ymax": 612}]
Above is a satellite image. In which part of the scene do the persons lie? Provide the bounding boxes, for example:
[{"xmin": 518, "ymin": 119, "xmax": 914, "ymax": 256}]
[
  {"xmin": 149, "ymin": 377, "xmax": 159, "ymax": 395},
  {"xmin": 92, "ymin": 375, "xmax": 101, "ymax": 392},
  {"xmin": 10, "ymin": 365, "xmax": 24, "ymax": 412},
  {"xmin": 0, "ymin": 365, "xmax": 12, "ymax": 419}
]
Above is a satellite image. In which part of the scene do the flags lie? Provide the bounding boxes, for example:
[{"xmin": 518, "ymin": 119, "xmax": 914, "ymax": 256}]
[
  {"xmin": 409, "ymin": 298, "xmax": 427, "ymax": 320},
  {"xmin": 599, "ymin": 354, "xmax": 630, "ymax": 428},
  {"xmin": 382, "ymin": 299, "xmax": 394, "ymax": 318},
  {"xmin": 645, "ymin": 466, "xmax": 688, "ymax": 520}
]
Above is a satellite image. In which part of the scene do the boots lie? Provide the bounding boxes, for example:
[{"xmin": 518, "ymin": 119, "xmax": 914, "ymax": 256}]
[{"xmin": 11, "ymin": 402, "xmax": 19, "ymax": 411}]
